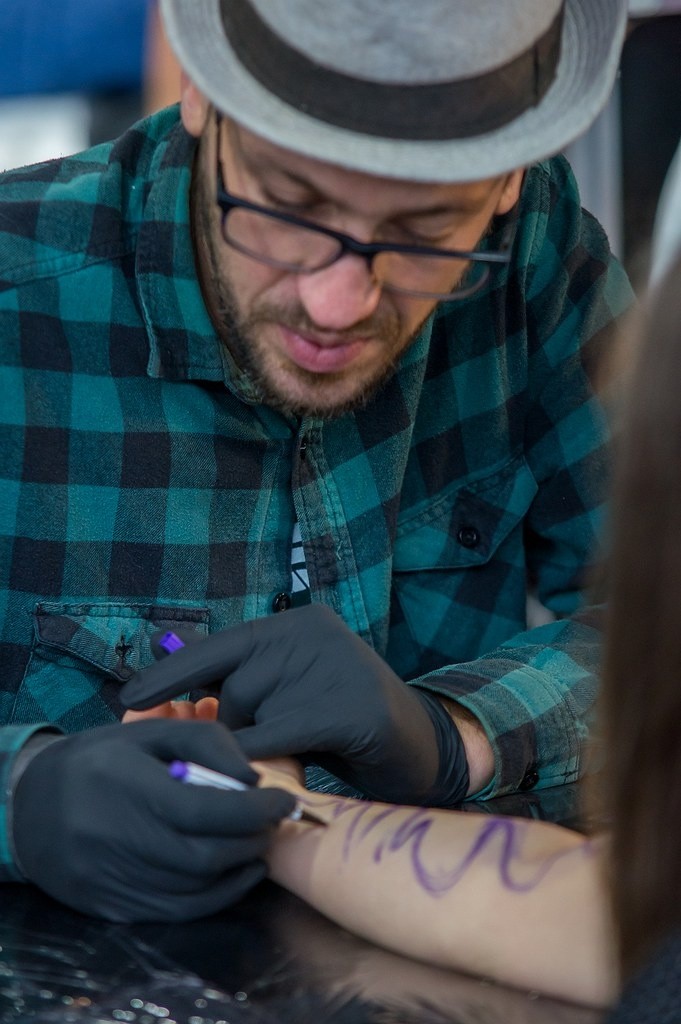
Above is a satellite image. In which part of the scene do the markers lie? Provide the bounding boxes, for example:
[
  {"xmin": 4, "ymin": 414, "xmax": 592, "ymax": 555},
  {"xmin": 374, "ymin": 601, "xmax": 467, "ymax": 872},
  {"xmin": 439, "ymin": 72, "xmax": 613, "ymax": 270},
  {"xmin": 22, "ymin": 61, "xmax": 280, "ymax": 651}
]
[{"xmin": 170, "ymin": 762, "xmax": 327, "ymax": 827}]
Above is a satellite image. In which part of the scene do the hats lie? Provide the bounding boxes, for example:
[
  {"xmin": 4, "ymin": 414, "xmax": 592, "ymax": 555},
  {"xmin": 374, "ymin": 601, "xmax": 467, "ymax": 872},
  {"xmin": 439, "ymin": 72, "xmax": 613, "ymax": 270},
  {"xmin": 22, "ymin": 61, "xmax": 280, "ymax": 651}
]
[{"xmin": 159, "ymin": 0, "xmax": 628, "ymax": 185}]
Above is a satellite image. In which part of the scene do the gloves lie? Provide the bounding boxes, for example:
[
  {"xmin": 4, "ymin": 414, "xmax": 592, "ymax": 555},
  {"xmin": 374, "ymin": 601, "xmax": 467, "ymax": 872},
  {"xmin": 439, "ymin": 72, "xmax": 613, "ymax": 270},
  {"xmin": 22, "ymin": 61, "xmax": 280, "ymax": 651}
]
[
  {"xmin": 11, "ymin": 719, "xmax": 296, "ymax": 926},
  {"xmin": 117, "ymin": 603, "xmax": 469, "ymax": 812}
]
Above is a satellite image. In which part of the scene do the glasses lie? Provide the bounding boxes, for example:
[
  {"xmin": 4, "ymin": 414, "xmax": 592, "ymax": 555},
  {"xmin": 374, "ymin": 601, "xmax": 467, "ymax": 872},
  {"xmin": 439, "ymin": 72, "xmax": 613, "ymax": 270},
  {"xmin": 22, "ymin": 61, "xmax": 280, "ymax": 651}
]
[{"xmin": 214, "ymin": 108, "xmax": 531, "ymax": 299}]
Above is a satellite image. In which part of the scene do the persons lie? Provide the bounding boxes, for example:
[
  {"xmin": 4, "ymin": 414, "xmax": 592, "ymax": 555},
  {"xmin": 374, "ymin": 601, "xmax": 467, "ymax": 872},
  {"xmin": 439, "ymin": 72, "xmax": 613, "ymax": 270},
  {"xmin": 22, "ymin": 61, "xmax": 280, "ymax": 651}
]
[
  {"xmin": 120, "ymin": 143, "xmax": 681, "ymax": 1024},
  {"xmin": 0, "ymin": 2, "xmax": 638, "ymax": 1024}
]
